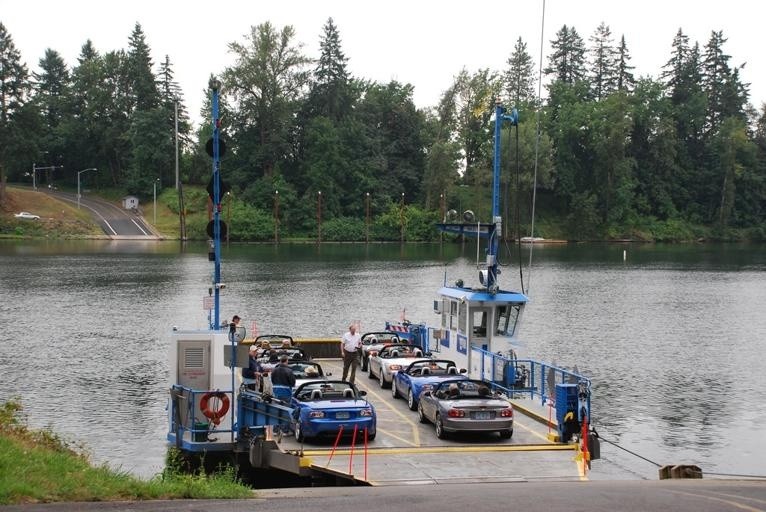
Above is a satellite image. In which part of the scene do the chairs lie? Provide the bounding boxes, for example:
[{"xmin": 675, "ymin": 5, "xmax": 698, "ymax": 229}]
[{"xmin": 262, "ymin": 336, "xmax": 489, "ymax": 401}]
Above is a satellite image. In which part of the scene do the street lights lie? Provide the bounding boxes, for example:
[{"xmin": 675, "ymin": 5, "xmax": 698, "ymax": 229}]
[{"xmin": 77, "ymin": 168, "xmax": 98, "ymax": 209}]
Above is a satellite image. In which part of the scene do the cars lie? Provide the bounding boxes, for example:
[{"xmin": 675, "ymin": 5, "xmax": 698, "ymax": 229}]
[{"xmin": 13, "ymin": 211, "xmax": 40, "ymax": 221}]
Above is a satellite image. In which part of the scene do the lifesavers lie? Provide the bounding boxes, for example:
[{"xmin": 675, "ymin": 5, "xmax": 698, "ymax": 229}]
[{"xmin": 199, "ymin": 392, "xmax": 229, "ymax": 417}]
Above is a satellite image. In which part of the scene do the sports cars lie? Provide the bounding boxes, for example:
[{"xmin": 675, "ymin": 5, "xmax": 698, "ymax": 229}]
[
  {"xmin": 391, "ymin": 359, "xmax": 469, "ymax": 411},
  {"xmin": 356, "ymin": 331, "xmax": 401, "ymax": 371},
  {"xmin": 252, "ymin": 334, "xmax": 336, "ymax": 399},
  {"xmin": 289, "ymin": 379, "xmax": 377, "ymax": 444},
  {"xmin": 366, "ymin": 344, "xmax": 433, "ymax": 389},
  {"xmin": 417, "ymin": 377, "xmax": 515, "ymax": 440}
]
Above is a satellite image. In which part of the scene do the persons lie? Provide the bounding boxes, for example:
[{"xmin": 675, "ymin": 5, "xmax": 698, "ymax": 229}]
[
  {"xmin": 257, "ymin": 338, "xmax": 293, "ymax": 372},
  {"xmin": 441, "ymin": 383, "xmax": 459, "ymax": 400},
  {"xmin": 228, "ymin": 315, "xmax": 242, "ymax": 337},
  {"xmin": 243, "ymin": 345, "xmax": 263, "ymax": 393},
  {"xmin": 339, "ymin": 325, "xmax": 363, "ymax": 385},
  {"xmin": 303, "ymin": 366, "xmax": 319, "ymax": 376},
  {"xmin": 271, "ymin": 355, "xmax": 296, "ymax": 438}
]
